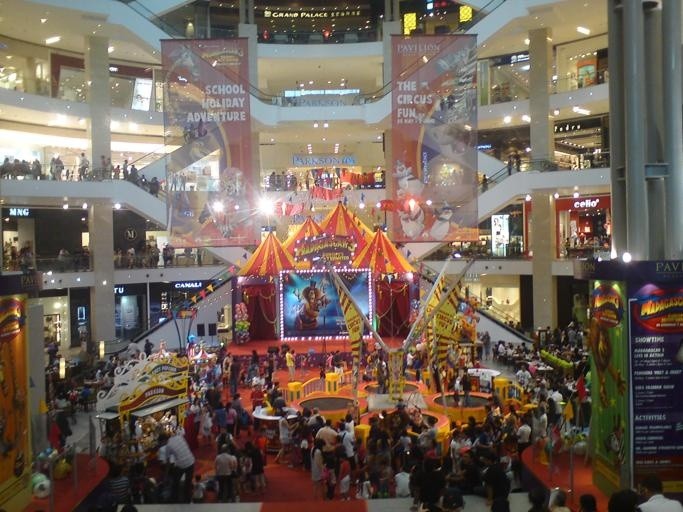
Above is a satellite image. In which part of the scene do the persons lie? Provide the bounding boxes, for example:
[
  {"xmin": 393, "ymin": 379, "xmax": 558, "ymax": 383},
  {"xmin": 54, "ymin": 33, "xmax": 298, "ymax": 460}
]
[{"xmin": 0, "ymin": 149, "xmax": 682, "ymax": 512}]
